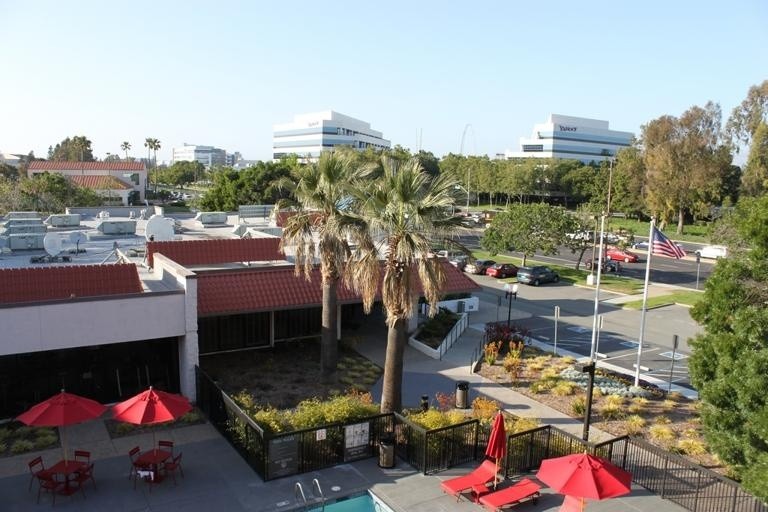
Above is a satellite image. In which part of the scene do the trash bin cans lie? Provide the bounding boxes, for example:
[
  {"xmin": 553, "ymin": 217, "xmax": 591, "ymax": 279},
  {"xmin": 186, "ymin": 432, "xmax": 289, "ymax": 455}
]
[
  {"xmin": 378, "ymin": 437, "xmax": 397, "ymax": 468},
  {"xmin": 455, "ymin": 380, "xmax": 470, "ymax": 409}
]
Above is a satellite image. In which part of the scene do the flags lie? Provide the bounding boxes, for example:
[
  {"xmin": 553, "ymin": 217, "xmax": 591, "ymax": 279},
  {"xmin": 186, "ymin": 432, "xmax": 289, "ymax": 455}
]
[{"xmin": 650, "ymin": 225, "xmax": 686, "ymax": 260}]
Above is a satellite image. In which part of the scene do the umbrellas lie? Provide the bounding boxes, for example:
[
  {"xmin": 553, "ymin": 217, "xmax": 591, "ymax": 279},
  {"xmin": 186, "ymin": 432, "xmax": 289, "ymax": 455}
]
[
  {"xmin": 14, "ymin": 389, "xmax": 109, "ymax": 496},
  {"xmin": 533, "ymin": 451, "xmax": 636, "ymax": 512},
  {"xmin": 484, "ymin": 408, "xmax": 510, "ymax": 493},
  {"xmin": 110, "ymin": 386, "xmax": 194, "ymax": 484}
]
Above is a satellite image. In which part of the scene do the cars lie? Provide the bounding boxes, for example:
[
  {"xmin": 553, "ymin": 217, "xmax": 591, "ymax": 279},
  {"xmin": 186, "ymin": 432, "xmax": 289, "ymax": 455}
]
[
  {"xmin": 450, "ymin": 208, "xmax": 501, "ymax": 229},
  {"xmin": 604, "ymin": 248, "xmax": 640, "ymax": 264},
  {"xmin": 426, "ymin": 247, "xmax": 522, "ymax": 279},
  {"xmin": 585, "ymin": 256, "xmax": 622, "ymax": 273},
  {"xmin": 170, "ymin": 191, "xmax": 206, "ymax": 203},
  {"xmin": 564, "ymin": 228, "xmax": 637, "ymax": 249}
]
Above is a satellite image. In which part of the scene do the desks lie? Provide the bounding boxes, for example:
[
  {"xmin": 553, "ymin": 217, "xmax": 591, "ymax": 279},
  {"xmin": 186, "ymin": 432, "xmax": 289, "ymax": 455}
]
[{"xmin": 470, "ymin": 484, "xmax": 489, "ymax": 504}]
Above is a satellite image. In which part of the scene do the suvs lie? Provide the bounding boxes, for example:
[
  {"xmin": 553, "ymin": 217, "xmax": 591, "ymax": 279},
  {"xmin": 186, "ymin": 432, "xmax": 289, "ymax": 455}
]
[
  {"xmin": 695, "ymin": 245, "xmax": 730, "ymax": 261},
  {"xmin": 517, "ymin": 265, "xmax": 558, "ymax": 286}
]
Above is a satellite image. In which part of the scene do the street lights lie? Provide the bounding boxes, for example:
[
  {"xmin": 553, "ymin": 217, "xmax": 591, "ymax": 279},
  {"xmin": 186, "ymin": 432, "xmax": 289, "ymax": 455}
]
[
  {"xmin": 607, "ymin": 156, "xmax": 617, "ymax": 213},
  {"xmin": 505, "ymin": 284, "xmax": 518, "ymax": 328},
  {"xmin": 106, "ymin": 152, "xmax": 111, "ymax": 207}
]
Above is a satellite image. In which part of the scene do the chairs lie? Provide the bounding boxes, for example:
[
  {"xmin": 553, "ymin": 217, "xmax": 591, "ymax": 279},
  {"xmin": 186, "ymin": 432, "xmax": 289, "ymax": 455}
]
[
  {"xmin": 27, "ymin": 450, "xmax": 99, "ymax": 507},
  {"xmin": 127, "ymin": 441, "xmax": 183, "ymax": 493},
  {"xmin": 478, "ymin": 478, "xmax": 542, "ymax": 512},
  {"xmin": 439, "ymin": 460, "xmax": 503, "ymax": 503}
]
